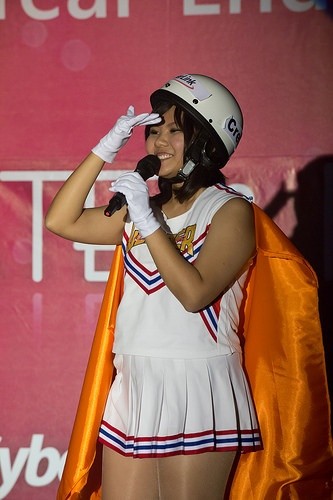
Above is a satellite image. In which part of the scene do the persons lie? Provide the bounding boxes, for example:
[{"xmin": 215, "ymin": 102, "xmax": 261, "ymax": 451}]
[{"xmin": 44, "ymin": 72, "xmax": 262, "ymax": 500}]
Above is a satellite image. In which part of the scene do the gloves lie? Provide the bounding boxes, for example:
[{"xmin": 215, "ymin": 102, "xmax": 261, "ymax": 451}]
[
  {"xmin": 90, "ymin": 106, "xmax": 162, "ymax": 163},
  {"xmin": 108, "ymin": 172, "xmax": 161, "ymax": 239}
]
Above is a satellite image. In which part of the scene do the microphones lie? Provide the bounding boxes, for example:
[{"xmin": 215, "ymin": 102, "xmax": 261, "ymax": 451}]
[{"xmin": 103, "ymin": 153, "xmax": 162, "ymax": 217}]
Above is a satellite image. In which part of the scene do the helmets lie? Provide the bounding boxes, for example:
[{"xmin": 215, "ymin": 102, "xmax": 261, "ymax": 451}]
[{"xmin": 150, "ymin": 74, "xmax": 243, "ymax": 159}]
